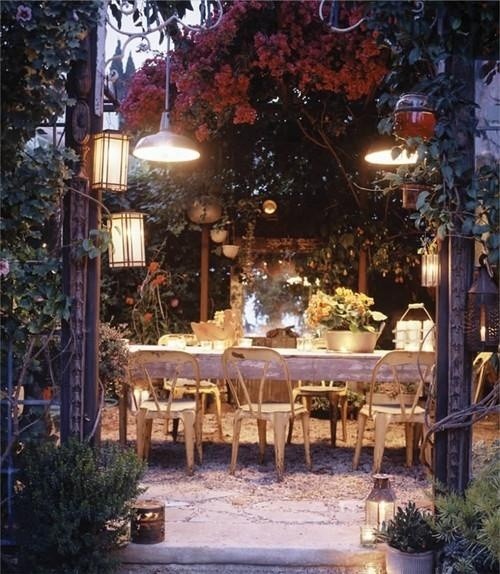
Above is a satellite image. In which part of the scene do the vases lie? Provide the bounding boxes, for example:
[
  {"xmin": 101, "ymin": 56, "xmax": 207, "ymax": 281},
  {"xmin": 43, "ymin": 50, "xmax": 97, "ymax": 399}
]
[{"xmin": 322, "ymin": 331, "xmax": 380, "ymax": 353}]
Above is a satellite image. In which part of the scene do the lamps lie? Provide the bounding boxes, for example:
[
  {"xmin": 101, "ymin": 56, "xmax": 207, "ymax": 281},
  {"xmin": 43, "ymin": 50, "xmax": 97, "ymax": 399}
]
[{"xmin": 87, "ymin": 24, "xmax": 202, "ymax": 270}]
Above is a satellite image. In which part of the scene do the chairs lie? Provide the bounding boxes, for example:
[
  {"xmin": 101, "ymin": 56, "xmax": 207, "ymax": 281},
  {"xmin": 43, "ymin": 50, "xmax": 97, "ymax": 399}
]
[
  {"xmin": 126, "ymin": 349, "xmax": 203, "ymax": 478},
  {"xmin": 158, "ymin": 336, "xmax": 224, "ymax": 444},
  {"xmin": 407, "ymin": 352, "xmax": 494, "ymax": 463},
  {"xmin": 220, "ymin": 347, "xmax": 311, "ymax": 482},
  {"xmin": 285, "ymin": 336, "xmax": 350, "ymax": 448},
  {"xmin": 351, "ymin": 351, "xmax": 439, "ymax": 477}
]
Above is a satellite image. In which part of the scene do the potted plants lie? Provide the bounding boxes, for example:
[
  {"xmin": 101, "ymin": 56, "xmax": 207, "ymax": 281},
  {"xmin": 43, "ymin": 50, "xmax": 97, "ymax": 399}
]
[{"xmin": 372, "ymin": 500, "xmax": 441, "ymax": 574}]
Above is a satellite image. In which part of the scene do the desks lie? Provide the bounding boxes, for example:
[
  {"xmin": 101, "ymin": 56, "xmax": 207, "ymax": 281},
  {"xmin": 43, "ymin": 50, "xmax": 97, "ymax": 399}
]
[{"xmin": 118, "ymin": 346, "xmax": 439, "ymax": 447}]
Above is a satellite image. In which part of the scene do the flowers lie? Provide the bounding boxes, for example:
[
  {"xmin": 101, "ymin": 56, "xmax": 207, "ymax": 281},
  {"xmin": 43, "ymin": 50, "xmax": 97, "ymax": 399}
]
[{"xmin": 303, "ymin": 287, "xmax": 388, "ymax": 337}]
